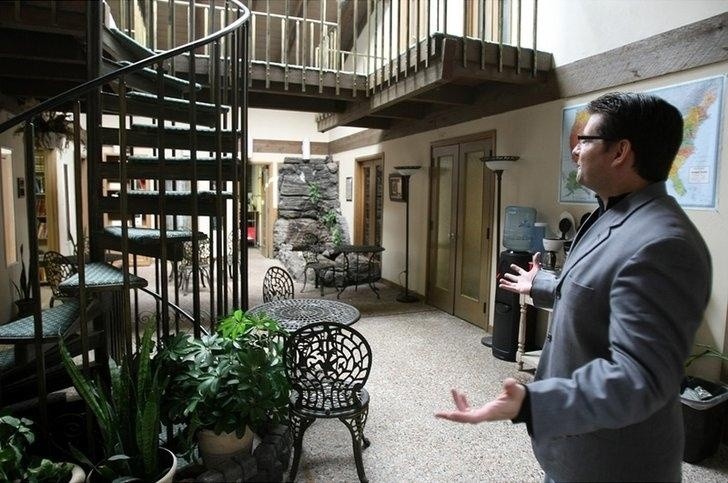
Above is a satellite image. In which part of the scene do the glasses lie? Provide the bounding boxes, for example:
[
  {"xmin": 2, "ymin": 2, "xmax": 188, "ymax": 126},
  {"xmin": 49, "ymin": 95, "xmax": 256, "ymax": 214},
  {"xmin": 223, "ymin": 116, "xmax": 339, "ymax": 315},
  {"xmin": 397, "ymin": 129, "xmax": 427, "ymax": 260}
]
[{"xmin": 575, "ymin": 132, "xmax": 616, "ymax": 148}]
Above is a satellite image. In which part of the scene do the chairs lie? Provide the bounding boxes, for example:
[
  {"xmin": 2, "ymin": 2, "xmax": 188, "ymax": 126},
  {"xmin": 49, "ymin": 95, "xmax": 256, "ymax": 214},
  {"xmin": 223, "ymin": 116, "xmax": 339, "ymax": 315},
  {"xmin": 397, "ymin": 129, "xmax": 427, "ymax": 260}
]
[
  {"xmin": 300, "ymin": 233, "xmax": 340, "ymax": 296},
  {"xmin": 43, "ymin": 251, "xmax": 77, "ymax": 308},
  {"xmin": 283, "ymin": 322, "xmax": 372, "ymax": 483},
  {"xmin": 263, "ymin": 267, "xmax": 294, "ymax": 303},
  {"xmin": 169, "ymin": 226, "xmax": 241, "ymax": 296}
]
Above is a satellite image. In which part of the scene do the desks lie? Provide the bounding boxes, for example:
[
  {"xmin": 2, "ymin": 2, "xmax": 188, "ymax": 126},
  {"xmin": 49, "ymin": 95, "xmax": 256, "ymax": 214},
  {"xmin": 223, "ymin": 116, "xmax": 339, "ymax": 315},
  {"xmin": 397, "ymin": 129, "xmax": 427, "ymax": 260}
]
[
  {"xmin": 245, "ymin": 299, "xmax": 361, "ymax": 377},
  {"xmin": 60, "ymin": 254, "xmax": 123, "ymax": 265},
  {"xmin": 333, "ymin": 245, "xmax": 385, "ymax": 299}
]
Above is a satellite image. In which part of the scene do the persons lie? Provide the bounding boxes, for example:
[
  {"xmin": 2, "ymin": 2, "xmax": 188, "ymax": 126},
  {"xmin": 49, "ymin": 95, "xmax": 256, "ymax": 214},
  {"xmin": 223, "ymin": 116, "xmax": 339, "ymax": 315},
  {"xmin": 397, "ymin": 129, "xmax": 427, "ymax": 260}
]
[{"xmin": 433, "ymin": 92, "xmax": 713, "ymax": 482}]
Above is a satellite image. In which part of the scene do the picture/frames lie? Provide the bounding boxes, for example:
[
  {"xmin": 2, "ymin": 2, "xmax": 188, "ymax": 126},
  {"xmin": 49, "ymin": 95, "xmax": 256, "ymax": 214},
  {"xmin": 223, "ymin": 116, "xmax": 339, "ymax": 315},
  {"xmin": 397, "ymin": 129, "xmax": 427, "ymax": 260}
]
[
  {"xmin": 388, "ymin": 173, "xmax": 406, "ymax": 202},
  {"xmin": 346, "ymin": 177, "xmax": 352, "ymax": 201}
]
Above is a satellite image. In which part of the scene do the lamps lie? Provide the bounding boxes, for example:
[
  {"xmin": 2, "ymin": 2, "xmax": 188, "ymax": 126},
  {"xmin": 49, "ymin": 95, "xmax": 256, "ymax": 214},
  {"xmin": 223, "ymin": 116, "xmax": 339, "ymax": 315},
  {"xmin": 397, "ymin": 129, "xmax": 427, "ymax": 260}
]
[
  {"xmin": 480, "ymin": 155, "xmax": 519, "ymax": 347},
  {"xmin": 394, "ymin": 166, "xmax": 422, "ymax": 303}
]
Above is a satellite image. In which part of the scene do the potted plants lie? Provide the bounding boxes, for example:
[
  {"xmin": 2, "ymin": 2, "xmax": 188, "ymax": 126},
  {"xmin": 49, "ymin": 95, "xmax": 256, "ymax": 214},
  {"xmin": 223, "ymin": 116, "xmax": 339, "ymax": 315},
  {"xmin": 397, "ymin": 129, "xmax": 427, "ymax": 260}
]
[{"xmin": 11, "ymin": 244, "xmax": 33, "ymax": 315}]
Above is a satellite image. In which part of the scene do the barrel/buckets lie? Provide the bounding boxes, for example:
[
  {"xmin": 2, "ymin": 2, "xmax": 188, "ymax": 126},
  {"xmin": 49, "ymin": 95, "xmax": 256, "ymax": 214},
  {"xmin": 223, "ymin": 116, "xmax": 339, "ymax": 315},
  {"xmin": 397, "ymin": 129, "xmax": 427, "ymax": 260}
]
[{"xmin": 504, "ymin": 205, "xmax": 535, "ymax": 252}]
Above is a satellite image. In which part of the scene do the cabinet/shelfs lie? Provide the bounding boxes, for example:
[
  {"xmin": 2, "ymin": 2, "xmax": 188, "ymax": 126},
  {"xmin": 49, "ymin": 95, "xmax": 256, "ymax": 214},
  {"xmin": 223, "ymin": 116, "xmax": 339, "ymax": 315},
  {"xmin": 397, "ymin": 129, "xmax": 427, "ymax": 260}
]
[{"xmin": 33, "ymin": 153, "xmax": 50, "ymax": 282}]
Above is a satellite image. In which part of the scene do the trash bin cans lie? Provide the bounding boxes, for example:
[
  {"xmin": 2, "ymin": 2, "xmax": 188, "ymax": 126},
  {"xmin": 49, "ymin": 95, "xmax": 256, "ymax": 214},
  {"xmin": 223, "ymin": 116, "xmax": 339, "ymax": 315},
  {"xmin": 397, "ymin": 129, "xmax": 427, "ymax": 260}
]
[{"xmin": 679, "ymin": 375, "xmax": 728, "ymax": 464}]
